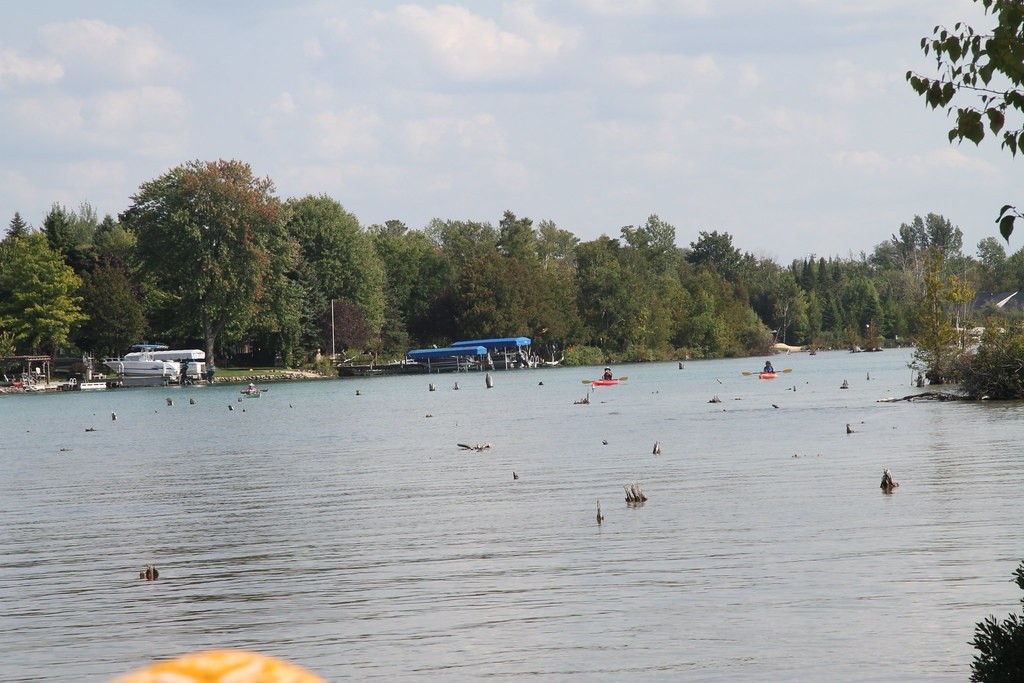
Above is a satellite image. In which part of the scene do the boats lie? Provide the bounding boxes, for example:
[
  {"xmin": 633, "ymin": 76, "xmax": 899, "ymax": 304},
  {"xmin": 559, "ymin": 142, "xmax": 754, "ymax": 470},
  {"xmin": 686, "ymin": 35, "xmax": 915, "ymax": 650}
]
[
  {"xmin": 759, "ymin": 369, "xmax": 778, "ymax": 379},
  {"xmin": 101, "ymin": 355, "xmax": 181, "ymax": 376},
  {"xmin": 242, "ymin": 392, "xmax": 261, "ymax": 399},
  {"xmin": 592, "ymin": 377, "xmax": 619, "ymax": 386}
]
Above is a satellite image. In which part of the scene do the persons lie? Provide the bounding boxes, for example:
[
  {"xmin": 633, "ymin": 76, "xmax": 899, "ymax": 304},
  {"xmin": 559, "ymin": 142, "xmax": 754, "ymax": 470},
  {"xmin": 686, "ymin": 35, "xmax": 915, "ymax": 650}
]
[
  {"xmin": 603, "ymin": 367, "xmax": 612, "ymax": 380},
  {"xmin": 760, "ymin": 361, "xmax": 776, "ymax": 374},
  {"xmin": 246, "ymin": 382, "xmax": 259, "ymax": 395}
]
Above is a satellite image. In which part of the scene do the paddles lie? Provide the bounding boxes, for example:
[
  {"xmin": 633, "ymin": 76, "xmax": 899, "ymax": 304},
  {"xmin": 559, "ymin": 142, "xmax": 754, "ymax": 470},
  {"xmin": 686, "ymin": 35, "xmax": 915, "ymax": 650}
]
[
  {"xmin": 742, "ymin": 368, "xmax": 792, "ymax": 376},
  {"xmin": 582, "ymin": 376, "xmax": 627, "ymax": 384},
  {"xmin": 240, "ymin": 387, "xmax": 268, "ymax": 394}
]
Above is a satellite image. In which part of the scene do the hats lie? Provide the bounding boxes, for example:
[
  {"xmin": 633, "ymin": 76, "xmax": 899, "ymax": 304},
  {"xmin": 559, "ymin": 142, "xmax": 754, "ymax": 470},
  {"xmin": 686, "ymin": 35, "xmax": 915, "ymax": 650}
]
[{"xmin": 605, "ymin": 367, "xmax": 611, "ymax": 371}]
